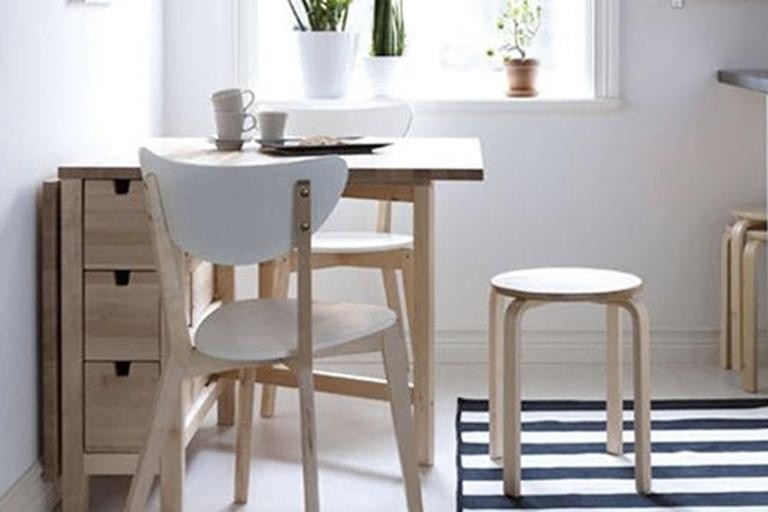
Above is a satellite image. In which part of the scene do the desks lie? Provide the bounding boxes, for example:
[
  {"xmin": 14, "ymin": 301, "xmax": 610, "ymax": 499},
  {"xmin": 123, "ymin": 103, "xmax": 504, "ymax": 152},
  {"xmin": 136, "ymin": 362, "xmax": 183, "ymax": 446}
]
[
  {"xmin": 717, "ymin": 69, "xmax": 768, "ymax": 94},
  {"xmin": 115, "ymin": 135, "xmax": 486, "ymax": 468}
]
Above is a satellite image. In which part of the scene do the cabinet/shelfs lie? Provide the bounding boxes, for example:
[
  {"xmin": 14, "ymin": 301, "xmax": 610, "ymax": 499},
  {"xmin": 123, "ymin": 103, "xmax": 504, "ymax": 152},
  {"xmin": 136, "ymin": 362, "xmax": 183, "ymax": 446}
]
[{"xmin": 58, "ymin": 156, "xmax": 218, "ymax": 512}]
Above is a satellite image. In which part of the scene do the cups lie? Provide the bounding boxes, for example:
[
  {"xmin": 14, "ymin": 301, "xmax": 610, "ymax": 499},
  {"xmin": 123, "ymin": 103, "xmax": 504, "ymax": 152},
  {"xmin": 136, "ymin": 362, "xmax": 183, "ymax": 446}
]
[
  {"xmin": 259, "ymin": 111, "xmax": 287, "ymax": 140},
  {"xmin": 210, "ymin": 88, "xmax": 255, "ymax": 141}
]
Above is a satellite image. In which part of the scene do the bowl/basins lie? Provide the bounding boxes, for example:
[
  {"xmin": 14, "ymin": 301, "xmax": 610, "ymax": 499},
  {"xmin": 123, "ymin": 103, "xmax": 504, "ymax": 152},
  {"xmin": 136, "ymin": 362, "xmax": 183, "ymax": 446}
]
[{"xmin": 213, "ymin": 138, "xmax": 246, "ymax": 152}]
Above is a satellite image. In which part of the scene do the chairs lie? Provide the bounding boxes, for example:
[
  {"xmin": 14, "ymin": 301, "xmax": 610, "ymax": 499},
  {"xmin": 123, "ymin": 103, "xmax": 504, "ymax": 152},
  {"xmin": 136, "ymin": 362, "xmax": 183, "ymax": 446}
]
[
  {"xmin": 260, "ymin": 97, "xmax": 420, "ymax": 420},
  {"xmin": 122, "ymin": 147, "xmax": 424, "ymax": 512}
]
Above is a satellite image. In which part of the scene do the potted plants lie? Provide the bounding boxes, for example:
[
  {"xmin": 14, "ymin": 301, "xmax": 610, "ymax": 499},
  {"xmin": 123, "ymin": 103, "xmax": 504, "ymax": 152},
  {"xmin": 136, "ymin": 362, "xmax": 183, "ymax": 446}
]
[
  {"xmin": 288, "ymin": 0, "xmax": 352, "ymax": 100},
  {"xmin": 365, "ymin": 0, "xmax": 407, "ymax": 99},
  {"xmin": 487, "ymin": 1, "xmax": 547, "ymax": 96}
]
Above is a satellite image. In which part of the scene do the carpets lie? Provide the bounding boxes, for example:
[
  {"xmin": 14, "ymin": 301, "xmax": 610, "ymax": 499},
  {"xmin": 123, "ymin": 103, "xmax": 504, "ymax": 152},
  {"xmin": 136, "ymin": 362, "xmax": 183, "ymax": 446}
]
[{"xmin": 456, "ymin": 397, "xmax": 768, "ymax": 509}]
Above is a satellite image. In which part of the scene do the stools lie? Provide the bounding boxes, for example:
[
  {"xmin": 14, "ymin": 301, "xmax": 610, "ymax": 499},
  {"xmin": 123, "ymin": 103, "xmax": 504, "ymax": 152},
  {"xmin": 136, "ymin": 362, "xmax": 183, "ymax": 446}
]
[
  {"xmin": 483, "ymin": 266, "xmax": 653, "ymax": 497},
  {"xmin": 718, "ymin": 211, "xmax": 768, "ymax": 393}
]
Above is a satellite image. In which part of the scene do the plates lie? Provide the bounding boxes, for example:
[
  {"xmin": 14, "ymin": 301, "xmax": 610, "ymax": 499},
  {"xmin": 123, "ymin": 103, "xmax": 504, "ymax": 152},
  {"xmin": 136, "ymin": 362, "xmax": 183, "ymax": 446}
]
[{"xmin": 254, "ymin": 138, "xmax": 397, "ymax": 156}]
